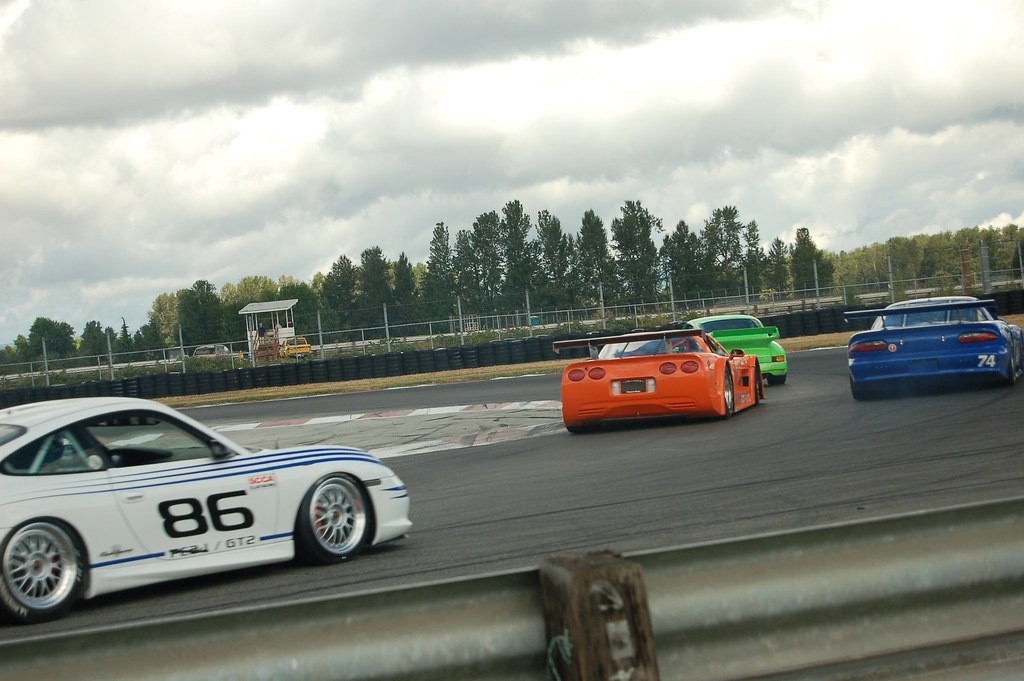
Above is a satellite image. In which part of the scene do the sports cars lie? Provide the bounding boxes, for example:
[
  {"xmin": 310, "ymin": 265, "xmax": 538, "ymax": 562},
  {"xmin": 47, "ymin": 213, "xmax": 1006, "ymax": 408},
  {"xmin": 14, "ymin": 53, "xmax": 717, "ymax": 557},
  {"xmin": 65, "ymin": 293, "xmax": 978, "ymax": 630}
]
[
  {"xmin": 669, "ymin": 314, "xmax": 789, "ymax": 384},
  {"xmin": 554, "ymin": 319, "xmax": 764, "ymax": 434},
  {"xmin": 842, "ymin": 295, "xmax": 1024, "ymax": 401},
  {"xmin": 0, "ymin": 397, "xmax": 413, "ymax": 628}
]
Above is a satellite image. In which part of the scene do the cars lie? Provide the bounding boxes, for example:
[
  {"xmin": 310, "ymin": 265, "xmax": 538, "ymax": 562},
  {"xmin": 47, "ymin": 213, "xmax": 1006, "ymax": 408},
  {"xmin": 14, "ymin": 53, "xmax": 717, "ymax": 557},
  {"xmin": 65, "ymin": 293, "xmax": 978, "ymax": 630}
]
[
  {"xmin": 278, "ymin": 336, "xmax": 312, "ymax": 359},
  {"xmin": 191, "ymin": 343, "xmax": 231, "ymax": 359}
]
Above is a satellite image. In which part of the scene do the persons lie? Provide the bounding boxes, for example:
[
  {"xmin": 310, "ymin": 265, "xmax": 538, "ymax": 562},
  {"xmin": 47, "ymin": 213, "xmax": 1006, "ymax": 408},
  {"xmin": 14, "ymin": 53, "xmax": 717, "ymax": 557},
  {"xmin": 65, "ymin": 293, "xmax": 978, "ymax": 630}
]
[
  {"xmin": 288, "ymin": 320, "xmax": 293, "ymax": 327},
  {"xmin": 275, "ymin": 321, "xmax": 282, "ymax": 329},
  {"xmin": 258, "ymin": 321, "xmax": 267, "ymax": 345}
]
